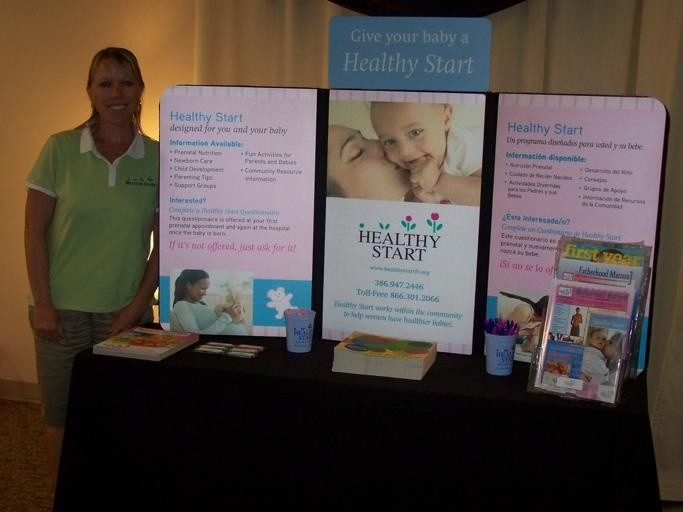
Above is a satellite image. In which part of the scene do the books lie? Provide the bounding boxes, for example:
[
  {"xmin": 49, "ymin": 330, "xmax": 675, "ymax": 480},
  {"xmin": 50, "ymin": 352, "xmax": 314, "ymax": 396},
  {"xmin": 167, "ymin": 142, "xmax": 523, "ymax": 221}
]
[
  {"xmin": 90, "ymin": 325, "xmax": 199, "ymax": 363},
  {"xmin": 329, "ymin": 330, "xmax": 437, "ymax": 383}
]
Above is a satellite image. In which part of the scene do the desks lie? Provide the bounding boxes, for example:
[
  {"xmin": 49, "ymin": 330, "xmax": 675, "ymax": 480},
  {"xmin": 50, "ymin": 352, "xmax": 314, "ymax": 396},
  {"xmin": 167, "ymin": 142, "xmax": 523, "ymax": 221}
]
[{"xmin": 52, "ymin": 315, "xmax": 662, "ymax": 512}]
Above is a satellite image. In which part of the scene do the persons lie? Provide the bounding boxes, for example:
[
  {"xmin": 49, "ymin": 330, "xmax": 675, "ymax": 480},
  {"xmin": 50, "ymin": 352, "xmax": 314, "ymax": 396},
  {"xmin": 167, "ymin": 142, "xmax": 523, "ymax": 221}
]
[
  {"xmin": 587, "ymin": 329, "xmax": 607, "ymax": 351},
  {"xmin": 368, "ymin": 101, "xmax": 480, "ymax": 207},
  {"xmin": 570, "ymin": 307, "xmax": 582, "ymax": 335},
  {"xmin": 170, "ymin": 266, "xmax": 245, "ymax": 334},
  {"xmin": 502, "ymin": 291, "xmax": 548, "ymax": 350},
  {"xmin": 325, "ymin": 124, "xmax": 418, "ymax": 199},
  {"xmin": 23, "ymin": 46, "xmax": 160, "ymax": 478},
  {"xmin": 601, "ymin": 332, "xmax": 621, "ymax": 367}
]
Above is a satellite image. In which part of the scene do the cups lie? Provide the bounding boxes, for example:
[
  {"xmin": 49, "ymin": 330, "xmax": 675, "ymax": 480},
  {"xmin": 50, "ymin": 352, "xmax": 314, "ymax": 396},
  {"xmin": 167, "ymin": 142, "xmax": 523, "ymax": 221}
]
[
  {"xmin": 283, "ymin": 308, "xmax": 319, "ymax": 354},
  {"xmin": 484, "ymin": 332, "xmax": 518, "ymax": 377}
]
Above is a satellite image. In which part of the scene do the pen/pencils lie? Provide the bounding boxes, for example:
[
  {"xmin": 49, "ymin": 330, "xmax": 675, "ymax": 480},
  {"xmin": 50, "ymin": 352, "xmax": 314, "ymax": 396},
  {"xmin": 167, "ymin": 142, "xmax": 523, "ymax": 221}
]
[
  {"xmin": 285, "ymin": 308, "xmax": 312, "ymax": 317},
  {"xmin": 194, "ymin": 341, "xmax": 264, "ymax": 359},
  {"xmin": 484, "ymin": 318, "xmax": 520, "ymax": 336}
]
